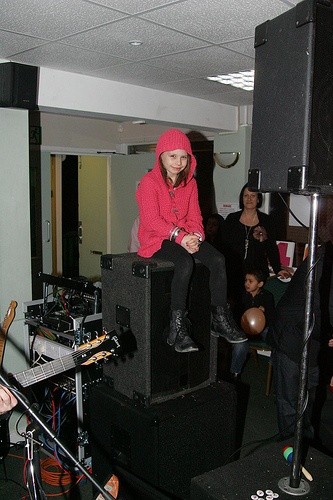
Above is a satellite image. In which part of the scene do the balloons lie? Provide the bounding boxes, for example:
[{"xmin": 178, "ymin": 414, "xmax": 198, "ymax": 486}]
[{"xmin": 240, "ymin": 307, "xmax": 266, "ymax": 335}]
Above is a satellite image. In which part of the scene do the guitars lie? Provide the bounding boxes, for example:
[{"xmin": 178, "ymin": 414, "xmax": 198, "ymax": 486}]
[{"xmin": 0, "ymin": 329, "xmax": 122, "ymax": 394}]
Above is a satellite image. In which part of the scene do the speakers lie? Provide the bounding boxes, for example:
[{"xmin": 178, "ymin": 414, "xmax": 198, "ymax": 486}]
[
  {"xmin": 99, "ymin": 250, "xmax": 218, "ymax": 408},
  {"xmin": 249, "ymin": 0, "xmax": 333, "ymax": 197},
  {"xmin": 0, "ymin": 62, "xmax": 38, "ymax": 110},
  {"xmin": 191, "ymin": 438, "xmax": 333, "ymax": 500},
  {"xmin": 89, "ymin": 373, "xmax": 233, "ymax": 500}
]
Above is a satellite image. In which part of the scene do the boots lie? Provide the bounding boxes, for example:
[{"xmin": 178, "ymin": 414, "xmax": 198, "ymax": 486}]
[
  {"xmin": 210, "ymin": 303, "xmax": 248, "ymax": 343},
  {"xmin": 166, "ymin": 308, "xmax": 199, "ymax": 353}
]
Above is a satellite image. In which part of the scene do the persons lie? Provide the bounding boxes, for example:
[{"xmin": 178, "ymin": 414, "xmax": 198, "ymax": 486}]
[
  {"xmin": 271, "ymin": 240, "xmax": 333, "ymax": 440},
  {"xmin": 224, "ymin": 182, "xmax": 291, "ymax": 324},
  {"xmin": 130, "ymin": 168, "xmax": 152, "ymax": 253},
  {"xmin": 205, "ymin": 213, "xmax": 224, "ymax": 244},
  {"xmin": 229, "ymin": 268, "xmax": 275, "ymax": 382},
  {"xmin": 137, "ymin": 129, "xmax": 248, "ymax": 352},
  {"xmin": 0, "ymin": 383, "xmax": 19, "ymax": 415}
]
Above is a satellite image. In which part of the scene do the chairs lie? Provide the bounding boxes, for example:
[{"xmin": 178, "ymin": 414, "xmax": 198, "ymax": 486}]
[{"xmin": 248, "ymin": 273, "xmax": 292, "ymax": 396}]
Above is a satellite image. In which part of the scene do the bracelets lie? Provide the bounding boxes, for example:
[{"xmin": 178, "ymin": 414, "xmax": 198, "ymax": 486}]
[{"xmin": 170, "ymin": 227, "xmax": 182, "ymax": 241}]
